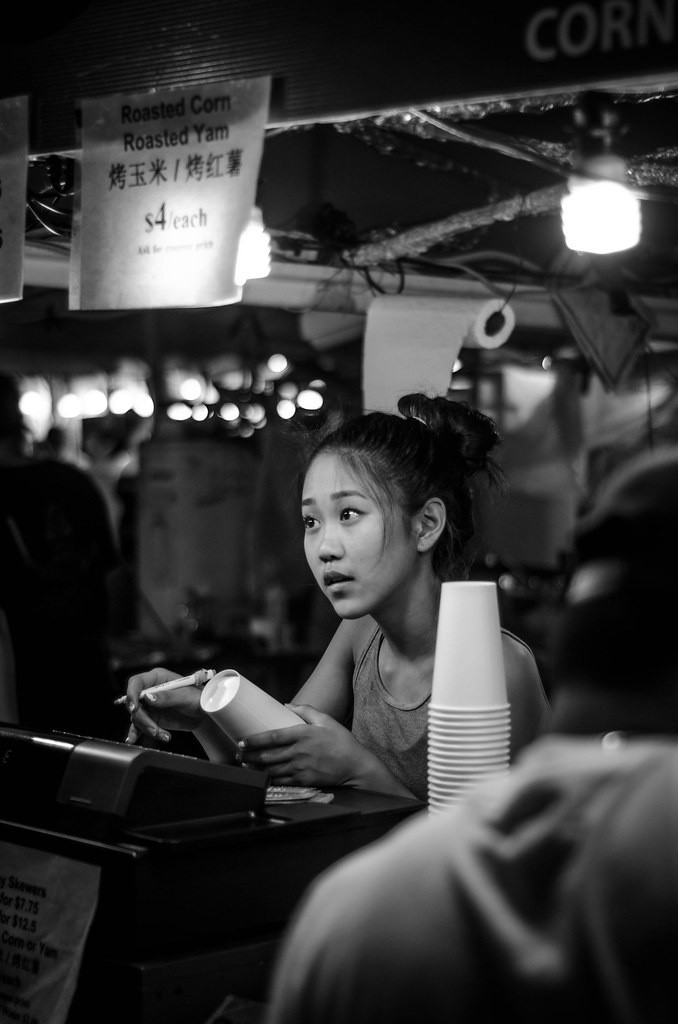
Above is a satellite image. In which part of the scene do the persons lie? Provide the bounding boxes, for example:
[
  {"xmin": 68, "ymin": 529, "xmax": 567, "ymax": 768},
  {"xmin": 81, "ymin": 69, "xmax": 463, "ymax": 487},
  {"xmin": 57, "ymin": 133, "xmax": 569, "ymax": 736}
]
[
  {"xmin": 0, "ymin": 368, "xmax": 121, "ymax": 698},
  {"xmin": 265, "ymin": 448, "xmax": 677, "ymax": 1023},
  {"xmin": 125, "ymin": 392, "xmax": 555, "ymax": 801}
]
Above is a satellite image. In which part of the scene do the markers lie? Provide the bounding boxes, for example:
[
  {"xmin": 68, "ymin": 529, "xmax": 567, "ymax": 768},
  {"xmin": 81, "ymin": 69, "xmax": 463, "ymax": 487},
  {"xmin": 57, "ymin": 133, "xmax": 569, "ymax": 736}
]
[{"xmin": 114, "ymin": 667, "xmax": 218, "ymax": 706}]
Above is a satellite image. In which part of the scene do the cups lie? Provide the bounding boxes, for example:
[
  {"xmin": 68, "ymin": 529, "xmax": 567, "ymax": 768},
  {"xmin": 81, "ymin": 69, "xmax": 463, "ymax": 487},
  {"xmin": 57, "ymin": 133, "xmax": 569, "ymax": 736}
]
[
  {"xmin": 199, "ymin": 668, "xmax": 309, "ymax": 765},
  {"xmin": 420, "ymin": 581, "xmax": 513, "ymax": 822}
]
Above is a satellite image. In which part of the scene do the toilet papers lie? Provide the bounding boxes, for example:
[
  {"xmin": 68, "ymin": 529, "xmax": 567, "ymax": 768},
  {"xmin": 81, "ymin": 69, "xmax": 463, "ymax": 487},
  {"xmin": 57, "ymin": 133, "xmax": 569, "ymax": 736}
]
[{"xmin": 361, "ymin": 296, "xmax": 517, "ymax": 417}]
